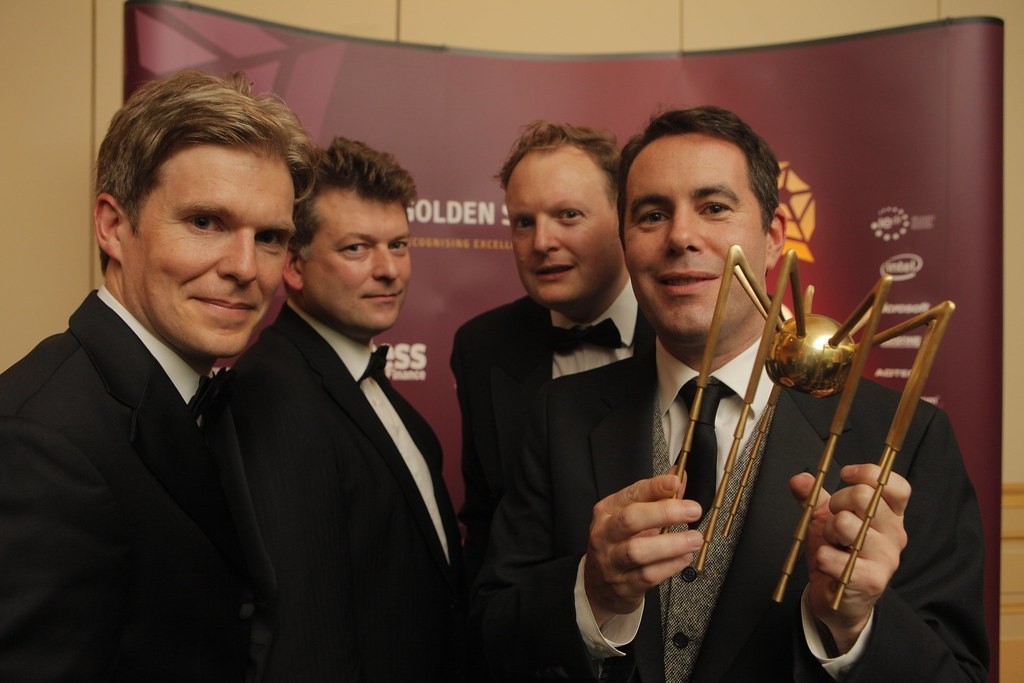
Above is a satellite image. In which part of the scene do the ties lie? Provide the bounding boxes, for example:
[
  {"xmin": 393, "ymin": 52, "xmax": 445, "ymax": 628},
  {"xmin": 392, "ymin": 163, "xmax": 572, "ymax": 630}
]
[{"xmin": 677, "ymin": 382, "xmax": 736, "ymax": 531}]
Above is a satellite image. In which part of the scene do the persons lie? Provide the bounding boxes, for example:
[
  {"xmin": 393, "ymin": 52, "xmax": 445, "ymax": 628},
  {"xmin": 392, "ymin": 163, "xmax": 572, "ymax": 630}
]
[
  {"xmin": 204, "ymin": 139, "xmax": 458, "ymax": 683},
  {"xmin": 451, "ymin": 123, "xmax": 656, "ymax": 683},
  {"xmin": 480, "ymin": 107, "xmax": 991, "ymax": 683},
  {"xmin": 0, "ymin": 69, "xmax": 315, "ymax": 683}
]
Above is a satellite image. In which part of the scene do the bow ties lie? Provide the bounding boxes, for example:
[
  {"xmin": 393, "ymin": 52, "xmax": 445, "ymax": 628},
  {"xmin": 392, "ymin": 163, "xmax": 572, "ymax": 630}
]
[
  {"xmin": 357, "ymin": 344, "xmax": 391, "ymax": 386},
  {"xmin": 549, "ymin": 317, "xmax": 622, "ymax": 359},
  {"xmin": 188, "ymin": 369, "xmax": 235, "ymax": 421}
]
